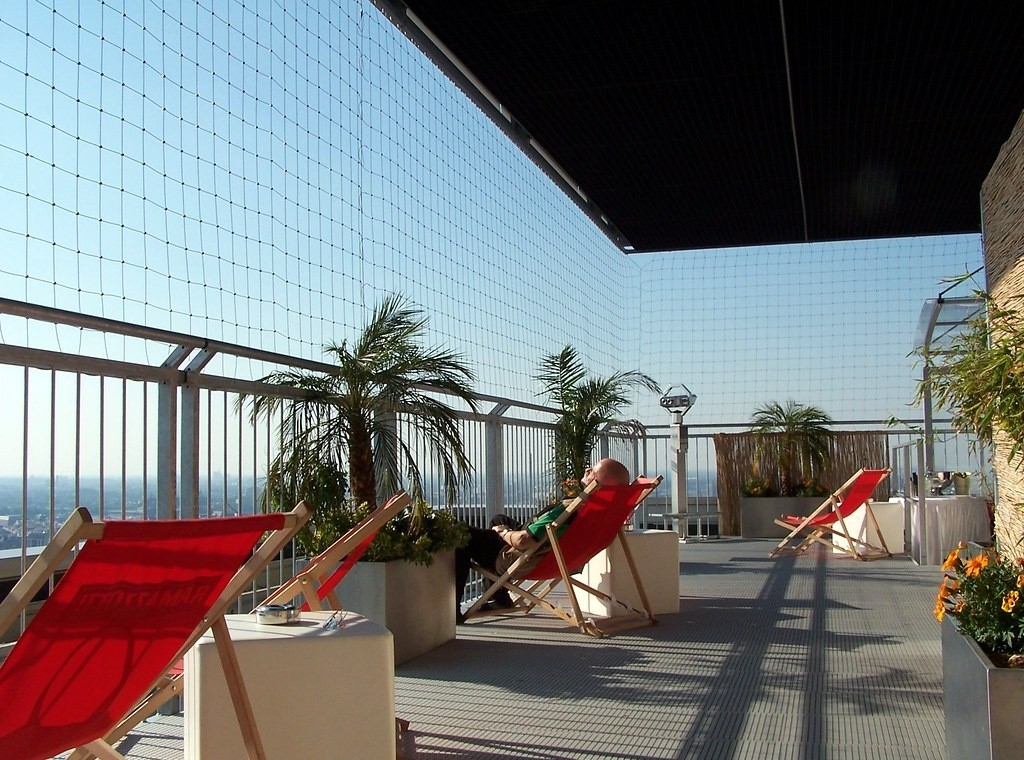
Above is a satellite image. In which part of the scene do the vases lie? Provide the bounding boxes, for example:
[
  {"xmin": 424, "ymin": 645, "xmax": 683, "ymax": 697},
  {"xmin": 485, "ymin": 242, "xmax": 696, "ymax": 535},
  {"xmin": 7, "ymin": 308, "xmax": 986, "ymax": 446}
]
[
  {"xmin": 740, "ymin": 498, "xmax": 831, "ymax": 538},
  {"xmin": 941, "ymin": 612, "xmax": 1024, "ymax": 760}
]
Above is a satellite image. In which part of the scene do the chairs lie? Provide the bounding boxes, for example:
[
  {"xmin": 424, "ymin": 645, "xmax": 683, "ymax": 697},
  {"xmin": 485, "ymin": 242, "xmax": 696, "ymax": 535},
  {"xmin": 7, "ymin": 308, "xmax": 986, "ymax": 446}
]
[
  {"xmin": 0, "ymin": 488, "xmax": 410, "ymax": 760},
  {"xmin": 768, "ymin": 468, "xmax": 893, "ymax": 561},
  {"xmin": 462, "ymin": 474, "xmax": 664, "ymax": 638}
]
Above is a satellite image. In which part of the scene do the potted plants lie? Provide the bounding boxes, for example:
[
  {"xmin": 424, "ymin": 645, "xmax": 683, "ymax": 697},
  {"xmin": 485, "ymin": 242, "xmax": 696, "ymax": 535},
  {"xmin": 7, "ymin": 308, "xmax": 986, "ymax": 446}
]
[{"xmin": 234, "ymin": 289, "xmax": 470, "ymax": 667}]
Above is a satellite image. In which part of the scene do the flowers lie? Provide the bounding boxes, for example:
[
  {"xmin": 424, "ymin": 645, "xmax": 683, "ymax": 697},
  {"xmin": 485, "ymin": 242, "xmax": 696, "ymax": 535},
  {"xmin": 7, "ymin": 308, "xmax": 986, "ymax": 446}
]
[
  {"xmin": 738, "ymin": 401, "xmax": 839, "ymax": 497},
  {"xmin": 933, "ymin": 470, "xmax": 1024, "ymax": 657},
  {"xmin": 532, "ymin": 342, "xmax": 664, "ymax": 503}
]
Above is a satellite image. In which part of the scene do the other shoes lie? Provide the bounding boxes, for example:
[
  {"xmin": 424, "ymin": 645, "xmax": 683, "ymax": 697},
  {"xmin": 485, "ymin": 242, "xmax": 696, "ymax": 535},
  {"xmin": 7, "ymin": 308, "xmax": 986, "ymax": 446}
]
[{"xmin": 478, "ymin": 593, "xmax": 515, "ymax": 612}]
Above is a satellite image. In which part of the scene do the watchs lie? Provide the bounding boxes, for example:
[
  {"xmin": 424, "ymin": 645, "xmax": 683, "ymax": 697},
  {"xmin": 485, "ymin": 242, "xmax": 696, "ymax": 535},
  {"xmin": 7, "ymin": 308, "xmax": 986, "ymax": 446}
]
[{"xmin": 501, "ymin": 528, "xmax": 513, "ymax": 538}]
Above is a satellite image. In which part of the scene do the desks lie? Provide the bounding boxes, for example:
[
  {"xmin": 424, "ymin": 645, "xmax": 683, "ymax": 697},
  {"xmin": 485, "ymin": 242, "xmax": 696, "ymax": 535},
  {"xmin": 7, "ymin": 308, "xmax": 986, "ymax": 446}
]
[
  {"xmin": 571, "ymin": 529, "xmax": 680, "ymax": 617},
  {"xmin": 185, "ymin": 610, "xmax": 396, "ymax": 760},
  {"xmin": 889, "ymin": 496, "xmax": 991, "ymax": 566},
  {"xmin": 832, "ymin": 502, "xmax": 905, "ymax": 554}
]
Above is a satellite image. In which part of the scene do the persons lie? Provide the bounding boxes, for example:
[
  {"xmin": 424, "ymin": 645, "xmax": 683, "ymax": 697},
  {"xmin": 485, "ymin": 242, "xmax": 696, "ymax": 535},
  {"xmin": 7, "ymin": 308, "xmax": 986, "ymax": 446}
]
[{"xmin": 456, "ymin": 458, "xmax": 630, "ymax": 624}]
